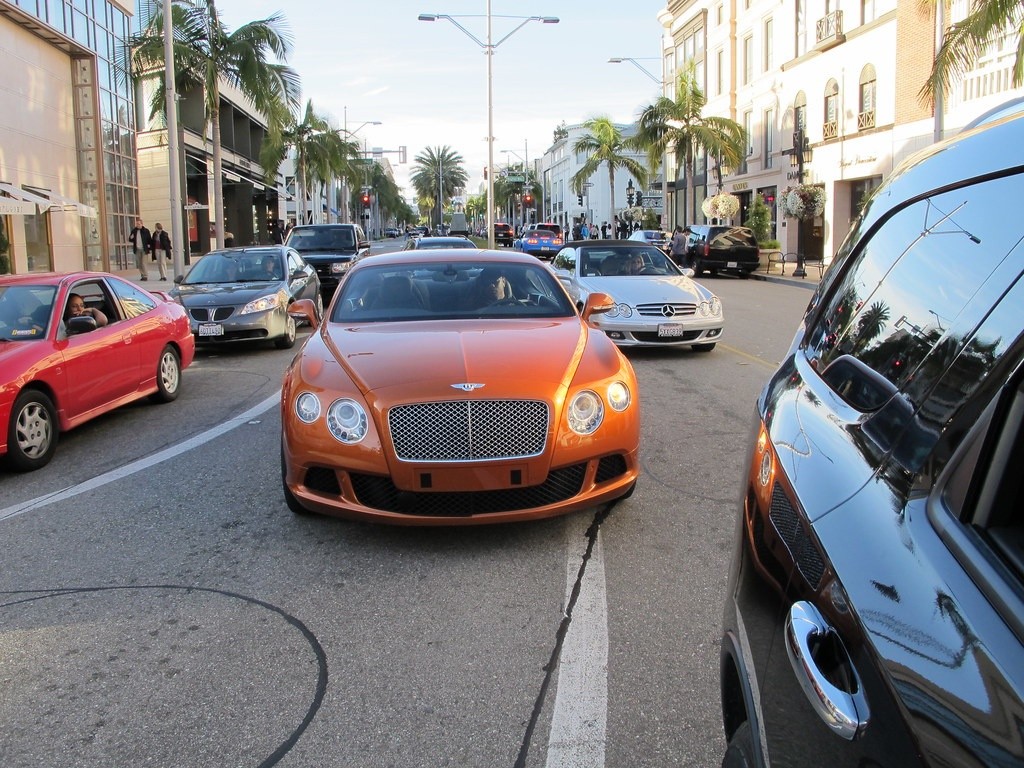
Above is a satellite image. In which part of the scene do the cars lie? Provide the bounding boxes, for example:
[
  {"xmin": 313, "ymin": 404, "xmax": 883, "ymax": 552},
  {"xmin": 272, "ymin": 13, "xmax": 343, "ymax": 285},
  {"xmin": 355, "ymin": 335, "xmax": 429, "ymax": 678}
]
[
  {"xmin": 384, "ymin": 226, "xmax": 430, "ymax": 238},
  {"xmin": 167, "ymin": 244, "xmax": 323, "ymax": 350},
  {"xmin": 513, "ymin": 230, "xmax": 563, "ymax": 259},
  {"xmin": 544, "ymin": 239, "xmax": 725, "ymax": 351},
  {"xmin": 627, "ymin": 230, "xmax": 673, "ymax": 250}
]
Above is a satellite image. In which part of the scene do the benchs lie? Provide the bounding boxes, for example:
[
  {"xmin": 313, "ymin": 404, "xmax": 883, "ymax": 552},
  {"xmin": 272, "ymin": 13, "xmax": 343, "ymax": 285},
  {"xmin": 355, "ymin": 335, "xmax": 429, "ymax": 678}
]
[
  {"xmin": 767, "ymin": 251, "xmax": 801, "ymax": 276},
  {"xmin": 801, "ymin": 255, "xmax": 829, "ymax": 281}
]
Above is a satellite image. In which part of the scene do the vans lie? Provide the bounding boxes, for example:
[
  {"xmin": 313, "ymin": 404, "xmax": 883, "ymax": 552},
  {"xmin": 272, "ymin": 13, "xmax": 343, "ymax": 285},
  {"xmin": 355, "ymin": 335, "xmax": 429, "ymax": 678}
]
[{"xmin": 662, "ymin": 225, "xmax": 760, "ymax": 278}]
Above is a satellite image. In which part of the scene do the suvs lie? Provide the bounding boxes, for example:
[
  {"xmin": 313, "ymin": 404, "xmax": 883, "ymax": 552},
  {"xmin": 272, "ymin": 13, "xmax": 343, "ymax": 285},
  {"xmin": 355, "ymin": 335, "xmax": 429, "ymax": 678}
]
[
  {"xmin": 717, "ymin": 107, "xmax": 1024, "ymax": 768},
  {"xmin": 528, "ymin": 218, "xmax": 563, "ymax": 239},
  {"xmin": 482, "ymin": 223, "xmax": 514, "ymax": 247},
  {"xmin": 284, "ymin": 223, "xmax": 370, "ymax": 306}
]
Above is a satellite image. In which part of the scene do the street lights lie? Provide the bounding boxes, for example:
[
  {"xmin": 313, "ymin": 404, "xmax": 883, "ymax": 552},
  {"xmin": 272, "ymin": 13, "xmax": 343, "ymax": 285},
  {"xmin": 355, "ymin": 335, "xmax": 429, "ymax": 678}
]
[
  {"xmin": 626, "ymin": 178, "xmax": 635, "ymax": 236},
  {"xmin": 790, "ymin": 128, "xmax": 813, "ymax": 276},
  {"xmin": 344, "ymin": 106, "xmax": 382, "ymax": 224},
  {"xmin": 417, "ymin": 0, "xmax": 560, "ymax": 248},
  {"xmin": 608, "ymin": 57, "xmax": 668, "ymax": 231}
]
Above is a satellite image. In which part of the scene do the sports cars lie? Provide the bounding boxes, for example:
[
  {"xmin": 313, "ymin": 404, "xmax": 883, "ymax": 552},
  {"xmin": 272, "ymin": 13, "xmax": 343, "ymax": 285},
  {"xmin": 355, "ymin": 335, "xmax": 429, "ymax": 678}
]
[
  {"xmin": 279, "ymin": 248, "xmax": 641, "ymax": 525},
  {"xmin": 0, "ymin": 271, "xmax": 195, "ymax": 471}
]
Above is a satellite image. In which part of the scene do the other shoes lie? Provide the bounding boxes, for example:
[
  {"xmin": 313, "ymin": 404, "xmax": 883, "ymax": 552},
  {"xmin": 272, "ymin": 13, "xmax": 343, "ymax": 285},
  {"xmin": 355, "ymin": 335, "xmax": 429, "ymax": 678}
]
[
  {"xmin": 160, "ymin": 277, "xmax": 166, "ymax": 281},
  {"xmin": 140, "ymin": 277, "xmax": 147, "ymax": 281}
]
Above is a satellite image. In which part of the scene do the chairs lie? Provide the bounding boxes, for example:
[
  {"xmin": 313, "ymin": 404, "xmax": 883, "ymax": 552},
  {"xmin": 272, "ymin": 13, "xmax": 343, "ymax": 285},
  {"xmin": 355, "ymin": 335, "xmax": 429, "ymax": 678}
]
[
  {"xmin": 369, "ymin": 275, "xmax": 422, "ymax": 312},
  {"xmin": 467, "ymin": 276, "xmax": 512, "ymax": 310}
]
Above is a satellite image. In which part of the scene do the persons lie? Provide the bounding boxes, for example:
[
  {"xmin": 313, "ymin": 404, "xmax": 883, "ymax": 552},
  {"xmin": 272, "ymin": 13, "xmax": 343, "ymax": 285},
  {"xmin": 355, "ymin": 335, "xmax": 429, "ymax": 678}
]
[
  {"xmin": 269, "ymin": 220, "xmax": 285, "ymax": 244},
  {"xmin": 62, "ymin": 293, "xmax": 106, "ymax": 328},
  {"xmin": 515, "ymin": 222, "xmax": 528, "ymax": 238},
  {"xmin": 669, "ymin": 225, "xmax": 693, "ymax": 268},
  {"xmin": 221, "ymin": 261, "xmax": 242, "ymax": 281},
  {"xmin": 601, "ymin": 221, "xmax": 612, "ymax": 239},
  {"xmin": 419, "ymin": 232, "xmax": 425, "ymax": 237},
  {"xmin": 286, "ymin": 222, "xmax": 293, "ymax": 240},
  {"xmin": 151, "ymin": 223, "xmax": 172, "ymax": 281},
  {"xmin": 460, "ymin": 267, "xmax": 507, "ymax": 311},
  {"xmin": 424, "ymin": 225, "xmax": 430, "ymax": 236},
  {"xmin": 224, "ymin": 227, "xmax": 236, "ymax": 248},
  {"xmin": 404, "ymin": 225, "xmax": 409, "ymax": 241},
  {"xmin": 129, "ymin": 218, "xmax": 152, "ymax": 281},
  {"xmin": 563, "ymin": 222, "xmax": 600, "ymax": 244},
  {"xmin": 253, "ymin": 255, "xmax": 281, "ymax": 279},
  {"xmin": 581, "ymin": 250, "xmax": 601, "ymax": 276},
  {"xmin": 620, "ymin": 255, "xmax": 645, "ymax": 274},
  {"xmin": 615, "ymin": 219, "xmax": 640, "ymax": 240}
]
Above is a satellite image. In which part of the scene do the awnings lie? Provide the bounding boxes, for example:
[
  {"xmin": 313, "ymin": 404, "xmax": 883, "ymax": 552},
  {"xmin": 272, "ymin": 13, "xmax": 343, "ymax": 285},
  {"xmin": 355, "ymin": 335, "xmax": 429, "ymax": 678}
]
[
  {"xmin": 186, "ymin": 153, "xmax": 297, "ymax": 202},
  {"xmin": 25, "ymin": 187, "xmax": 97, "ymax": 218},
  {"xmin": 0, "ymin": 183, "xmax": 68, "ymax": 215}
]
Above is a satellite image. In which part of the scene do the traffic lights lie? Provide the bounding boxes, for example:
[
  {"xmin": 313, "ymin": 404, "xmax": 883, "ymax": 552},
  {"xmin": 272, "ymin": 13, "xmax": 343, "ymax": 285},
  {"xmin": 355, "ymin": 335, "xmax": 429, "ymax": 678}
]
[
  {"xmin": 636, "ymin": 191, "xmax": 643, "ymax": 206},
  {"xmin": 484, "ymin": 167, "xmax": 487, "ymax": 179},
  {"xmin": 577, "ymin": 194, "xmax": 583, "ymax": 207},
  {"xmin": 524, "ymin": 196, "xmax": 532, "ymax": 207},
  {"xmin": 362, "ymin": 195, "xmax": 370, "ymax": 209}
]
